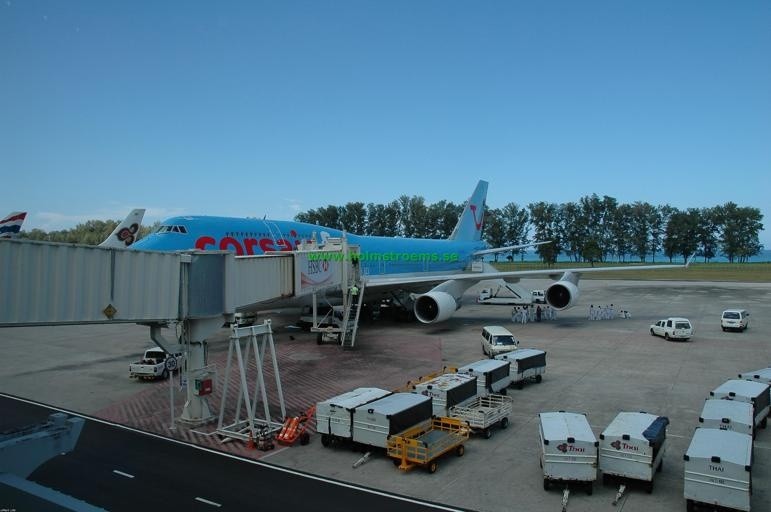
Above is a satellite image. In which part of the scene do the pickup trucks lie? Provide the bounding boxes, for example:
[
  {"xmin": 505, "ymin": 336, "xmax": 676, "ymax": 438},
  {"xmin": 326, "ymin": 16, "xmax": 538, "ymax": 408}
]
[{"xmin": 129, "ymin": 347, "xmax": 187, "ymax": 380}]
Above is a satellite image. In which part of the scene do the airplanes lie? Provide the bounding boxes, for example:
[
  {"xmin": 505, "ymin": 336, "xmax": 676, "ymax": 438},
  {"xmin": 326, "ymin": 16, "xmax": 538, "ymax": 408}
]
[{"xmin": 96, "ymin": 181, "xmax": 695, "ymax": 345}]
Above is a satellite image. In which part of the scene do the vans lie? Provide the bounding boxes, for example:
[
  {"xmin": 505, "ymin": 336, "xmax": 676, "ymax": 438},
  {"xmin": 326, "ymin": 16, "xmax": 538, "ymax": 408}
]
[
  {"xmin": 480, "ymin": 326, "xmax": 520, "ymax": 359},
  {"xmin": 720, "ymin": 309, "xmax": 750, "ymax": 333},
  {"xmin": 649, "ymin": 318, "xmax": 694, "ymax": 341}
]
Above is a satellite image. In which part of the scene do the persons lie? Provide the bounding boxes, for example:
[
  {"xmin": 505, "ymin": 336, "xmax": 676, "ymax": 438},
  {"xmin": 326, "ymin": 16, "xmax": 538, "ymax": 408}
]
[
  {"xmin": 350, "ymin": 285, "xmax": 359, "ymax": 305},
  {"xmin": 587, "ymin": 304, "xmax": 631, "ymax": 320},
  {"xmin": 512, "ymin": 305, "xmax": 557, "ymax": 325}
]
